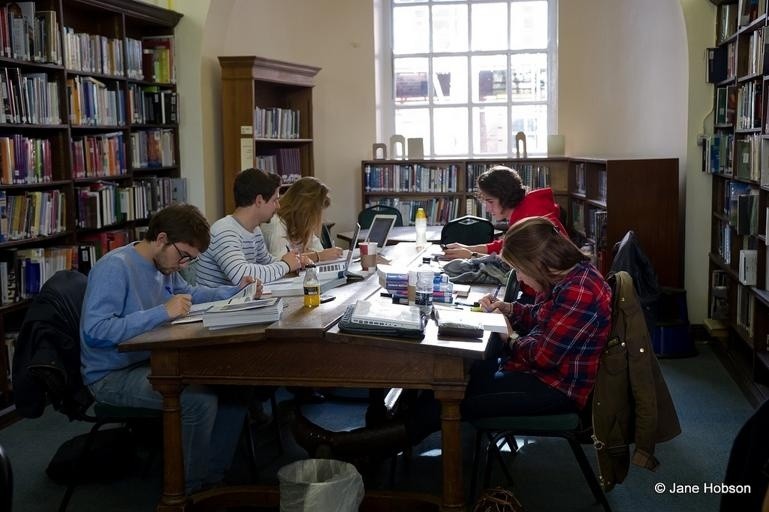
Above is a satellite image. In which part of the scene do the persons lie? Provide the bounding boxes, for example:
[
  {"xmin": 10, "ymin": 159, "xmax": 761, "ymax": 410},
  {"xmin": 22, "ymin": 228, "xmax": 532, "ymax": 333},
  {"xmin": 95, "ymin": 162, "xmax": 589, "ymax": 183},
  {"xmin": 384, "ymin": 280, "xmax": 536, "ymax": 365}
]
[
  {"xmin": 439, "ymin": 166, "xmax": 569, "ymax": 266},
  {"xmin": 262, "ymin": 177, "xmax": 343, "ymax": 260},
  {"xmin": 367, "ymin": 216, "xmax": 613, "ymax": 472},
  {"xmin": 78, "ymin": 203, "xmax": 263, "ymax": 486},
  {"xmin": 186, "ymin": 165, "xmax": 315, "ymax": 288}
]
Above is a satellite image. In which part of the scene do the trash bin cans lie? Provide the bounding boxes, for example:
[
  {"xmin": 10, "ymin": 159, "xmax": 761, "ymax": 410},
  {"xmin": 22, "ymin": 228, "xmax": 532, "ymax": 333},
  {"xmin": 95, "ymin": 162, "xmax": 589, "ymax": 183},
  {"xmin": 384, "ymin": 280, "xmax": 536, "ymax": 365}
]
[
  {"xmin": 285, "ymin": 244, "xmax": 290, "ymax": 252},
  {"xmin": 332, "ymin": 239, "xmax": 334, "ymax": 248},
  {"xmin": 454, "ymin": 302, "xmax": 474, "ymax": 306},
  {"xmin": 486, "ymin": 284, "xmax": 502, "ymax": 313},
  {"xmin": 164, "ymin": 286, "xmax": 190, "ymax": 315},
  {"xmin": 276, "ymin": 459, "xmax": 364, "ymax": 512}
]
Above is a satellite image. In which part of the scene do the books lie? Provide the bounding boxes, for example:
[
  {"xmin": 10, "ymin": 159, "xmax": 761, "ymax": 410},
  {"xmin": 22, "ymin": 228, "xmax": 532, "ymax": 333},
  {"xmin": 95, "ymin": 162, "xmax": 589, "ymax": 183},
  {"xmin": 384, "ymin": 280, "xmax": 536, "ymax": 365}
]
[
  {"xmin": 432, "ymin": 298, "xmax": 507, "ymax": 338},
  {"xmin": 0, "ymin": 190, "xmax": 66, "ymax": 242},
  {"xmin": 740, "ymin": 0, "xmax": 766, "ymax": 29},
  {"xmin": 252, "ymin": 106, "xmax": 300, "ymax": 137},
  {"xmin": 71, "ymin": 129, "xmax": 128, "ymax": 178},
  {"xmin": 1, "ymin": 332, "xmax": 21, "ymax": 385},
  {"xmin": 170, "ymin": 281, "xmax": 284, "ymax": 332},
  {"xmin": 132, "ymin": 175, "xmax": 188, "ymax": 219},
  {"xmin": 74, "ymin": 181, "xmax": 134, "ymax": 230},
  {"xmin": 718, "ymin": 179, "xmax": 758, "ymax": 236},
  {"xmin": 77, "ymin": 227, "xmax": 149, "ymax": 273},
  {"xmin": 62, "ymin": 24, "xmax": 124, "ymax": 78},
  {"xmin": 705, "ymin": 40, "xmax": 735, "ymax": 84},
  {"xmin": 734, "ymin": 135, "xmax": 762, "ymax": 183},
  {"xmin": 0, "ymin": 1, "xmax": 62, "ymax": 65},
  {"xmin": 465, "ymin": 198, "xmax": 493, "ymax": 221},
  {"xmin": 718, "ymin": 3, "xmax": 738, "ymax": 43},
  {"xmin": 128, "ymin": 80, "xmax": 178, "ymax": 125},
  {"xmin": 701, "ymin": 134, "xmax": 733, "ymax": 177},
  {"xmin": 715, "ymin": 86, "xmax": 736, "ymax": 125},
  {"xmin": 715, "ymin": 220, "xmax": 759, "ymax": 285},
  {"xmin": 575, "ymin": 164, "xmax": 585, "ymax": 193},
  {"xmin": 451, "ymin": 284, "xmax": 471, "ymax": 297},
  {"xmin": 0, "ymin": 245, "xmax": 80, "ymax": 305},
  {"xmin": 1, "ymin": 66, "xmax": 64, "ymax": 125},
  {"xmin": 256, "ymin": 148, "xmax": 305, "ymax": 182},
  {"xmin": 337, "ymin": 296, "xmax": 424, "ymax": 339},
  {"xmin": 129, "ymin": 128, "xmax": 177, "ymax": 170},
  {"xmin": 377, "ymin": 262, "xmax": 453, "ymax": 304},
  {"xmin": 748, "ymin": 26, "xmax": 769, "ymax": 76},
  {"xmin": 468, "ymin": 163, "xmax": 550, "ymax": 197},
  {"xmin": 125, "ymin": 31, "xmax": 176, "ymax": 83},
  {"xmin": 705, "ymin": 271, "xmax": 756, "ymax": 346},
  {"xmin": 597, "ymin": 169, "xmax": 607, "ymax": 200},
  {"xmin": 67, "ymin": 73, "xmax": 126, "ymax": 128},
  {"xmin": 270, "ymin": 269, "xmax": 347, "ymax": 297},
  {"xmin": 572, "ymin": 201, "xmax": 584, "ymax": 232},
  {"xmin": 588, "ymin": 206, "xmax": 608, "ymax": 244},
  {"xmin": 365, "ymin": 197, "xmax": 460, "ymax": 229},
  {"xmin": 736, "ymin": 81, "xmax": 766, "ymax": 130},
  {"xmin": 0, "ymin": 133, "xmax": 52, "ymax": 185},
  {"xmin": 366, "ymin": 164, "xmax": 461, "ymax": 191}
]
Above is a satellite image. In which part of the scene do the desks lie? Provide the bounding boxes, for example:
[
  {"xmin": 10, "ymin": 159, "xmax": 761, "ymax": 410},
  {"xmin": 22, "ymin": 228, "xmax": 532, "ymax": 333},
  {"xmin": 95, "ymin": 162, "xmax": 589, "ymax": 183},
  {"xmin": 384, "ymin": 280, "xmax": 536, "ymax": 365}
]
[
  {"xmin": 336, "ymin": 225, "xmax": 504, "ymax": 247},
  {"xmin": 118, "ymin": 242, "xmax": 504, "ymax": 512}
]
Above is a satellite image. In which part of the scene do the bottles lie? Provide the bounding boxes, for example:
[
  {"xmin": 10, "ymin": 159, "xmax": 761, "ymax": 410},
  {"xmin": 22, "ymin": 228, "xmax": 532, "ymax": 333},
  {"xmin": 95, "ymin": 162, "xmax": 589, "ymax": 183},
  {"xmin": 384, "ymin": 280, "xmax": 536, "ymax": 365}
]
[
  {"xmin": 415, "ymin": 257, "xmax": 435, "ymax": 316},
  {"xmin": 415, "ymin": 208, "xmax": 427, "ymax": 252},
  {"xmin": 303, "ymin": 263, "xmax": 320, "ymax": 308}
]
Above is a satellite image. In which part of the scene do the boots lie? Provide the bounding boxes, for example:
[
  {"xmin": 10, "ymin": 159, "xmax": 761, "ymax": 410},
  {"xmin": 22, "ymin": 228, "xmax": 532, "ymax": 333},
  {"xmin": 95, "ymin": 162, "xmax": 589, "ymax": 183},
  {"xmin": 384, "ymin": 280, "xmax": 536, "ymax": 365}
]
[{"xmin": 296, "ymin": 415, "xmax": 405, "ymax": 456}]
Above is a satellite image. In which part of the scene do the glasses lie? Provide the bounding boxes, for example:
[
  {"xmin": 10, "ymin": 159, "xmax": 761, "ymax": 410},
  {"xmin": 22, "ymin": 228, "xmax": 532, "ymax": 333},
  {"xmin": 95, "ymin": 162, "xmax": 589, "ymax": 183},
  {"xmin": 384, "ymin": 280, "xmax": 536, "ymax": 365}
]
[{"xmin": 173, "ymin": 242, "xmax": 199, "ymax": 265}]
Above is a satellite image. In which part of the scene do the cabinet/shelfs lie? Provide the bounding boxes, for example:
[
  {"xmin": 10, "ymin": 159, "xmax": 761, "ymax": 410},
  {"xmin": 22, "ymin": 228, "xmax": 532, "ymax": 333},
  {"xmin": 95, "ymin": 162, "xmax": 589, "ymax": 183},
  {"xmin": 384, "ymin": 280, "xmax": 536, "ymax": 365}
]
[
  {"xmin": 361, "ymin": 156, "xmax": 679, "ymax": 293},
  {"xmin": 707, "ymin": 0, "xmax": 767, "ymax": 409},
  {"xmin": 216, "ymin": 56, "xmax": 321, "ymax": 215},
  {"xmin": 2, "ymin": 0, "xmax": 183, "ymax": 426}
]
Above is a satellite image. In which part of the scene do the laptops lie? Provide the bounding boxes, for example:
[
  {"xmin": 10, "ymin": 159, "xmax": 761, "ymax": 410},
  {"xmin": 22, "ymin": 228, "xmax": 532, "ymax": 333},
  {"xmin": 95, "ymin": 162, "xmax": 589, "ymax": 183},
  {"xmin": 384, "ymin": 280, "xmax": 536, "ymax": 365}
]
[
  {"xmin": 318, "ymin": 223, "xmax": 360, "ymax": 279},
  {"xmin": 339, "ymin": 216, "xmax": 396, "ymax": 256},
  {"xmin": 351, "ymin": 300, "xmax": 420, "ymax": 330}
]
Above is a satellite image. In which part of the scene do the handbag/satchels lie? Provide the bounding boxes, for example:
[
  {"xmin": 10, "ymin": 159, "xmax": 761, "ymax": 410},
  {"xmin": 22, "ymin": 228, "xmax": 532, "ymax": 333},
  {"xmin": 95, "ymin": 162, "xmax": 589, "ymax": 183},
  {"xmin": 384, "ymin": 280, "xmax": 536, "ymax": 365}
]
[{"xmin": 49, "ymin": 428, "xmax": 138, "ymax": 486}]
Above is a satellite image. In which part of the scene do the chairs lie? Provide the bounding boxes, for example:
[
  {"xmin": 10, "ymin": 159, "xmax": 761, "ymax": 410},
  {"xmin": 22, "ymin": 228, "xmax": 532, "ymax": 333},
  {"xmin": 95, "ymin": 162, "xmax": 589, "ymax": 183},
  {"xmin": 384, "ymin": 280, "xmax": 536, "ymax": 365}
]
[
  {"xmin": 469, "ymin": 271, "xmax": 627, "ymax": 512},
  {"xmin": 32, "ymin": 270, "xmax": 162, "ymax": 511},
  {"xmin": 358, "ymin": 205, "xmax": 403, "ymax": 229},
  {"xmin": 441, "ymin": 215, "xmax": 494, "ymax": 245}
]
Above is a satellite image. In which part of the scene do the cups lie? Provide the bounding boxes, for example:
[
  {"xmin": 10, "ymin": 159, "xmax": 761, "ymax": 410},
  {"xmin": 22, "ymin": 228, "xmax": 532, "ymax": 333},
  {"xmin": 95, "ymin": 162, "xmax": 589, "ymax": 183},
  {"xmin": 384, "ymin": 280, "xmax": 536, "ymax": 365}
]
[{"xmin": 360, "ymin": 242, "xmax": 380, "ymax": 272}]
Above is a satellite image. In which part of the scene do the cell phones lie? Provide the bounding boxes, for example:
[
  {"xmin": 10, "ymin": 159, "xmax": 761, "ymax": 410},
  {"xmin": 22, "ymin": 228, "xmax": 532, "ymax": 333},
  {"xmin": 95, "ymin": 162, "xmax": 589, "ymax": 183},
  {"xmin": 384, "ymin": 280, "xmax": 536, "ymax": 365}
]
[{"xmin": 319, "ymin": 294, "xmax": 336, "ymax": 303}]
